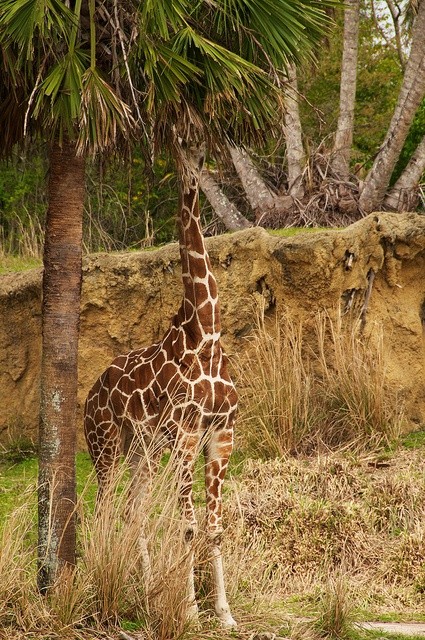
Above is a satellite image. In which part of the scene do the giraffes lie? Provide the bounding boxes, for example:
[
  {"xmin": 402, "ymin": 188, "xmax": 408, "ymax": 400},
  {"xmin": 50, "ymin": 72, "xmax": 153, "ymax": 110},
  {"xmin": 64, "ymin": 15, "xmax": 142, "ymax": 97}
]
[{"xmin": 84, "ymin": 124, "xmax": 240, "ymax": 640}]
